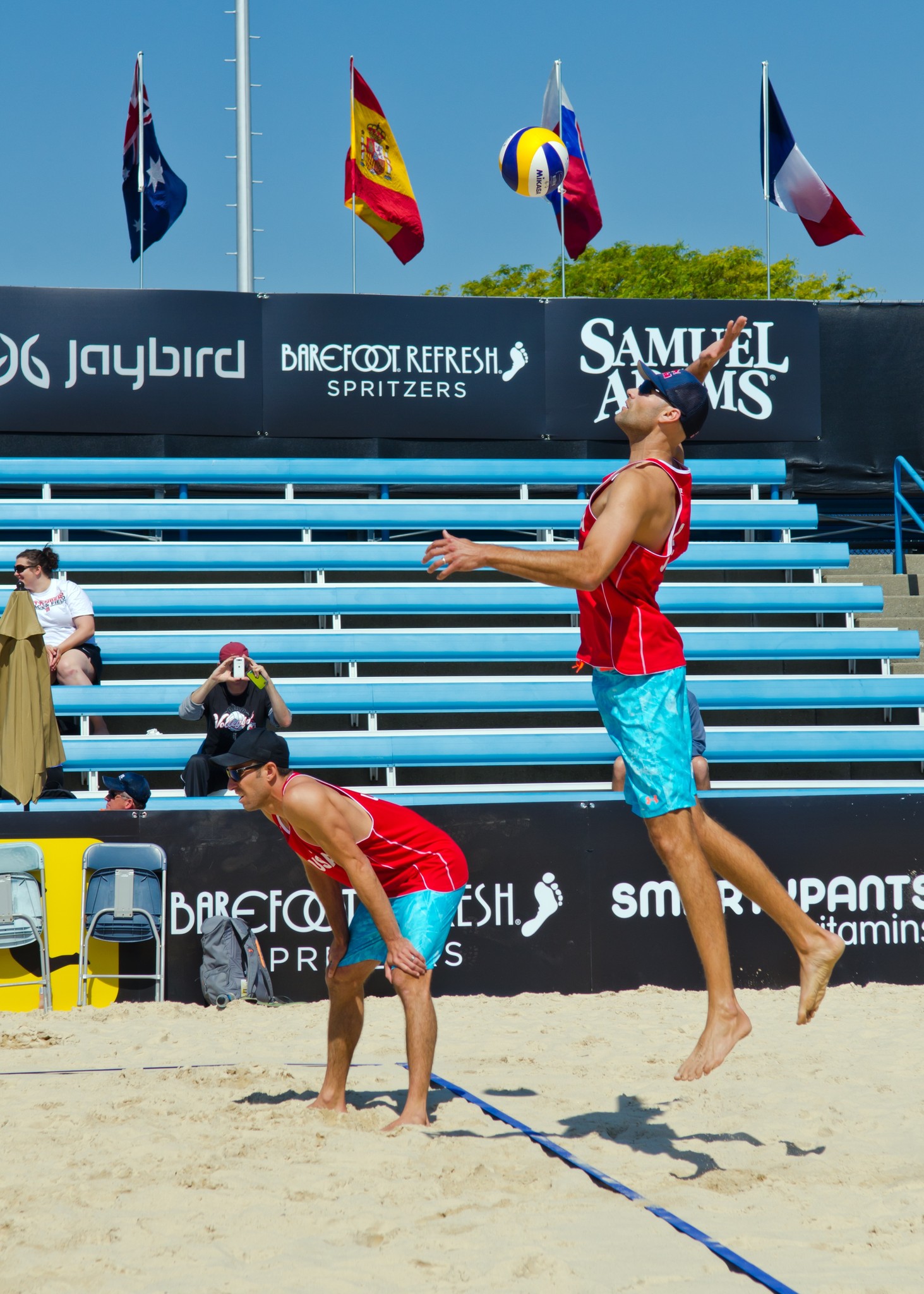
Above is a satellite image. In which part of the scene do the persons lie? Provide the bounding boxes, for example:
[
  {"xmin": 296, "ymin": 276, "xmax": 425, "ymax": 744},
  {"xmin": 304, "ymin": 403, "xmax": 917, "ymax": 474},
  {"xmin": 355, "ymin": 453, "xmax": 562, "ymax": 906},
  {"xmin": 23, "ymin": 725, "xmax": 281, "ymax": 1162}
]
[
  {"xmin": 209, "ymin": 727, "xmax": 469, "ymax": 1135},
  {"xmin": 611, "ymin": 687, "xmax": 711, "ymax": 793},
  {"xmin": 14, "ymin": 546, "xmax": 110, "ymax": 735},
  {"xmin": 101, "ymin": 772, "xmax": 150, "ymax": 811},
  {"xmin": 420, "ymin": 316, "xmax": 846, "ymax": 1082},
  {"xmin": 177, "ymin": 641, "xmax": 292, "ymax": 798}
]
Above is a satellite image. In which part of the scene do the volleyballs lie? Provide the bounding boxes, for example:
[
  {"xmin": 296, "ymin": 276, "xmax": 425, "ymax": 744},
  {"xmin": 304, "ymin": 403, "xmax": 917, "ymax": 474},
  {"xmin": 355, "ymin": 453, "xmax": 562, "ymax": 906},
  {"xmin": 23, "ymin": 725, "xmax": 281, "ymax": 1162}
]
[{"xmin": 498, "ymin": 126, "xmax": 569, "ymax": 197}]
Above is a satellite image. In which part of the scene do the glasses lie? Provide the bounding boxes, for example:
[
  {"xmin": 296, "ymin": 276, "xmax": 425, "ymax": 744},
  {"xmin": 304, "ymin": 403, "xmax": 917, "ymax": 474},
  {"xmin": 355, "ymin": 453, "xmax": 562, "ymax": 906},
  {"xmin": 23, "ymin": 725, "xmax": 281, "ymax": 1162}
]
[
  {"xmin": 107, "ymin": 790, "xmax": 135, "ymax": 804},
  {"xmin": 226, "ymin": 763, "xmax": 265, "ymax": 782},
  {"xmin": 638, "ymin": 379, "xmax": 688, "ymax": 419},
  {"xmin": 14, "ymin": 564, "xmax": 38, "ymax": 573}
]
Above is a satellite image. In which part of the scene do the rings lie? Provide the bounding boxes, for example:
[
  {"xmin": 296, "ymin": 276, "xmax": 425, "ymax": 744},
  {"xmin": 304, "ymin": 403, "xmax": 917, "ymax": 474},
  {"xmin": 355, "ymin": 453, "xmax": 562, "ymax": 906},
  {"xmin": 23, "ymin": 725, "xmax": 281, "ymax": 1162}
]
[
  {"xmin": 441, "ymin": 555, "xmax": 447, "ymax": 564},
  {"xmin": 411, "ymin": 956, "xmax": 415, "ymax": 963}
]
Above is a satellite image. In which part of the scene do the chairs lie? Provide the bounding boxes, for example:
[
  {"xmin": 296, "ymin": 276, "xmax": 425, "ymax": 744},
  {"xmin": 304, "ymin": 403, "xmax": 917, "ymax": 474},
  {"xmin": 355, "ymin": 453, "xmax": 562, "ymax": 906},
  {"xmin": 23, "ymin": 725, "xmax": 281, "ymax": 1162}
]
[
  {"xmin": 77, "ymin": 842, "xmax": 168, "ymax": 1005},
  {"xmin": 0, "ymin": 842, "xmax": 54, "ymax": 1011}
]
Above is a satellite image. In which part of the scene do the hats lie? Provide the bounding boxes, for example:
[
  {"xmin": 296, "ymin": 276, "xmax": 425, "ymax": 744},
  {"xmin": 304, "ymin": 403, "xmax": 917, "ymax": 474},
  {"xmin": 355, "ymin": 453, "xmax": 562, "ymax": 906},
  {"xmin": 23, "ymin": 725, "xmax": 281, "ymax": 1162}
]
[
  {"xmin": 219, "ymin": 642, "xmax": 249, "ymax": 662},
  {"xmin": 209, "ymin": 728, "xmax": 290, "ymax": 770},
  {"xmin": 637, "ymin": 358, "xmax": 710, "ymax": 439},
  {"xmin": 102, "ymin": 772, "xmax": 151, "ymax": 805}
]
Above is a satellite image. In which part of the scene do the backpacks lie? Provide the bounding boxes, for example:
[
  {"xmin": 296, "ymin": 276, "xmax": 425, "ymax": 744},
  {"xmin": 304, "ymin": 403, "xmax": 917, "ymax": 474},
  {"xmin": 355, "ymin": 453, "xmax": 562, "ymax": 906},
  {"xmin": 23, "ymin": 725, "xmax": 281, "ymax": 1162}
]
[{"xmin": 199, "ymin": 914, "xmax": 295, "ymax": 1007}]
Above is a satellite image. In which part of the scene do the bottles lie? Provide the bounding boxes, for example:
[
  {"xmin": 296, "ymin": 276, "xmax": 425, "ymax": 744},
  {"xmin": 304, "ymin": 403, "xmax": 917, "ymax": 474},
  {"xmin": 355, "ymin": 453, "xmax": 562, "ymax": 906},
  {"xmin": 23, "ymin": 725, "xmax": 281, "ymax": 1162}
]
[{"xmin": 215, "ymin": 993, "xmax": 236, "ymax": 1008}]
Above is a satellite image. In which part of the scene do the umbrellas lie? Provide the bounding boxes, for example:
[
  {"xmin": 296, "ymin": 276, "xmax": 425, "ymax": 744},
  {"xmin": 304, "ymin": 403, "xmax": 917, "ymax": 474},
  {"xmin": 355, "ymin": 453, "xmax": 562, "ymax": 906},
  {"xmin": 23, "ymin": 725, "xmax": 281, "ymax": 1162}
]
[{"xmin": 0, "ymin": 581, "xmax": 68, "ymax": 811}]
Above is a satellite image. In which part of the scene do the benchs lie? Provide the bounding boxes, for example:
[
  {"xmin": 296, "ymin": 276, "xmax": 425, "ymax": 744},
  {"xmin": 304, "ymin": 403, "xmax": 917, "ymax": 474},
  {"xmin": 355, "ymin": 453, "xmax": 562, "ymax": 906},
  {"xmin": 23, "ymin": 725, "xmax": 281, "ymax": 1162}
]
[{"xmin": 0, "ymin": 456, "xmax": 924, "ymax": 812}]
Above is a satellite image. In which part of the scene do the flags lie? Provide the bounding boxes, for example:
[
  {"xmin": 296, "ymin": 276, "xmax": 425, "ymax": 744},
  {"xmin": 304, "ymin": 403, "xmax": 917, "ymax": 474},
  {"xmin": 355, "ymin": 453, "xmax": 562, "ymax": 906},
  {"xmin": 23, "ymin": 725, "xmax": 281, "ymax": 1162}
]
[
  {"xmin": 122, "ymin": 49, "xmax": 188, "ymax": 264},
  {"xmin": 343, "ymin": 53, "xmax": 425, "ymax": 266},
  {"xmin": 537, "ymin": 60, "xmax": 603, "ymax": 263},
  {"xmin": 759, "ymin": 59, "xmax": 866, "ymax": 247}
]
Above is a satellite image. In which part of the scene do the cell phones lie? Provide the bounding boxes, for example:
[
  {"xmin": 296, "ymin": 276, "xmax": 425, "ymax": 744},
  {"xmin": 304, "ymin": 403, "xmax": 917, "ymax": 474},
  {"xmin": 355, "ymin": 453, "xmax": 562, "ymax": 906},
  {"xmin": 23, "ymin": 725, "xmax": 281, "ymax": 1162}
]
[{"xmin": 233, "ymin": 657, "xmax": 244, "ymax": 678}]
